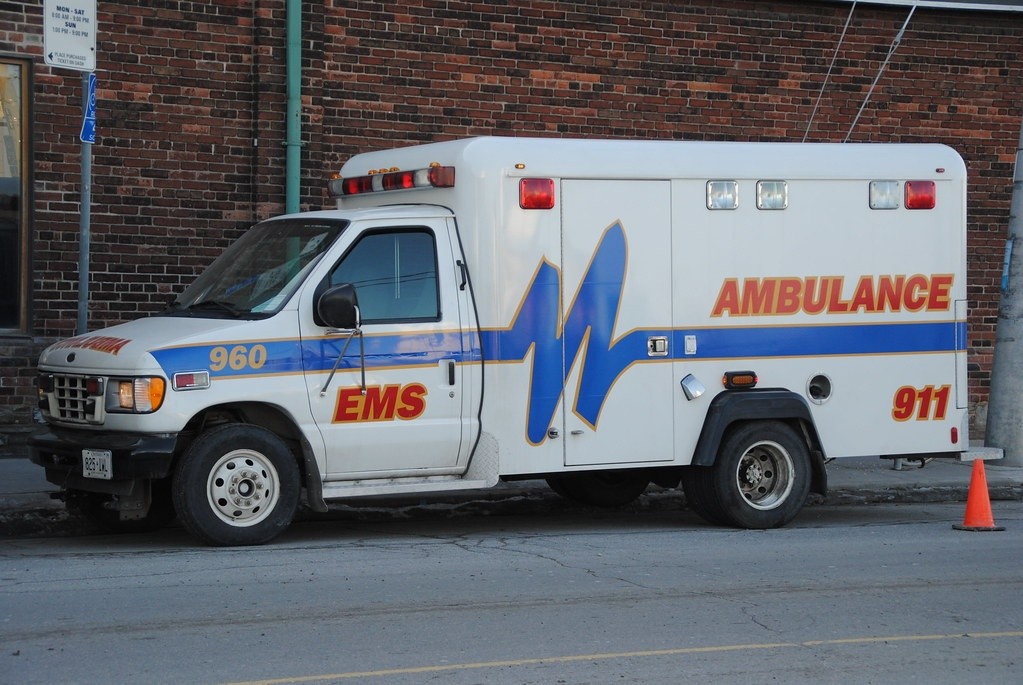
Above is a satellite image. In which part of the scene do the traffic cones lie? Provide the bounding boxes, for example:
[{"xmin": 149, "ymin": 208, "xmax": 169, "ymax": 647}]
[{"xmin": 952, "ymin": 456, "xmax": 1008, "ymax": 534}]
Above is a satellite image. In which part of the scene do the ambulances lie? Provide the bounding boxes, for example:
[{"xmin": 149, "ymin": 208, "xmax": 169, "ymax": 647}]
[{"xmin": 21, "ymin": 138, "xmax": 1003, "ymax": 545}]
